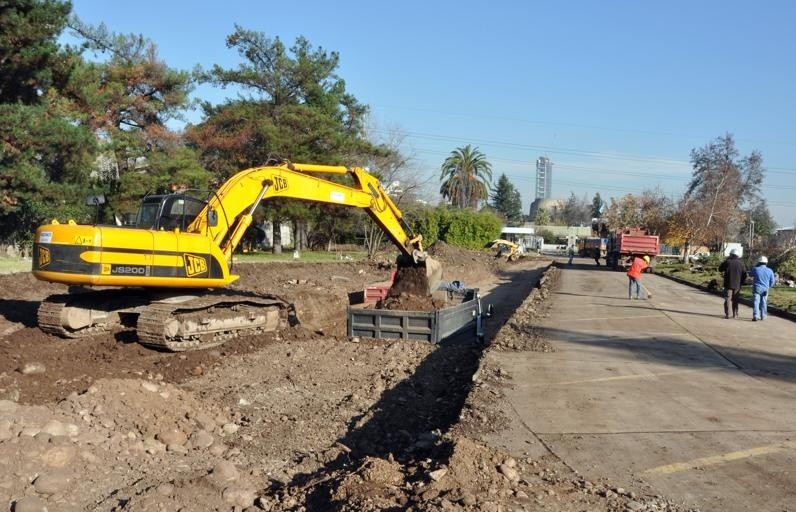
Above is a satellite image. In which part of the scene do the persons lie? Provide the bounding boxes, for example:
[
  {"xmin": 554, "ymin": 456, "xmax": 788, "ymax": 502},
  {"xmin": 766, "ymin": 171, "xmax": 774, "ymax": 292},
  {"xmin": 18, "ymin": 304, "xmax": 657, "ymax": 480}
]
[
  {"xmin": 566, "ymin": 244, "xmax": 575, "ymax": 266},
  {"xmin": 624, "ymin": 254, "xmax": 651, "ymax": 300},
  {"xmin": 718, "ymin": 249, "xmax": 748, "ymax": 320},
  {"xmin": 593, "ymin": 244, "xmax": 601, "ymax": 267},
  {"xmin": 748, "ymin": 255, "xmax": 776, "ymax": 321}
]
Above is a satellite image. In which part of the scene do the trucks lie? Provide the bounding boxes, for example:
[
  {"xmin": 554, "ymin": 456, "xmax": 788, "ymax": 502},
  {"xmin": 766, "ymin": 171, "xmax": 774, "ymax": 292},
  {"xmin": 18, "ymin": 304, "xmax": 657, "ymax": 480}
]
[{"xmin": 605, "ymin": 226, "xmax": 662, "ymax": 274}]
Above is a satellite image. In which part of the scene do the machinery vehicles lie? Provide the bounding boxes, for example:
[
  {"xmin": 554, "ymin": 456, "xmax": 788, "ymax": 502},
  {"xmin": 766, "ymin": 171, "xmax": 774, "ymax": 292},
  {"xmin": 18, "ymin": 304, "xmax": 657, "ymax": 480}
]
[
  {"xmin": 486, "ymin": 239, "xmax": 524, "ymax": 262},
  {"xmin": 27, "ymin": 164, "xmax": 444, "ymax": 354},
  {"xmin": 575, "ymin": 237, "xmax": 609, "ymax": 259}
]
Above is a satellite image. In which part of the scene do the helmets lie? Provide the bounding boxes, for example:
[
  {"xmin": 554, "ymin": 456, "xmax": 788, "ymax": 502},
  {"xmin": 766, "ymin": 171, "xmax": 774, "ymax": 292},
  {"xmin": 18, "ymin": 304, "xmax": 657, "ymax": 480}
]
[
  {"xmin": 730, "ymin": 249, "xmax": 740, "ymax": 256},
  {"xmin": 643, "ymin": 255, "xmax": 650, "ymax": 264},
  {"xmin": 758, "ymin": 255, "xmax": 768, "ymax": 263}
]
